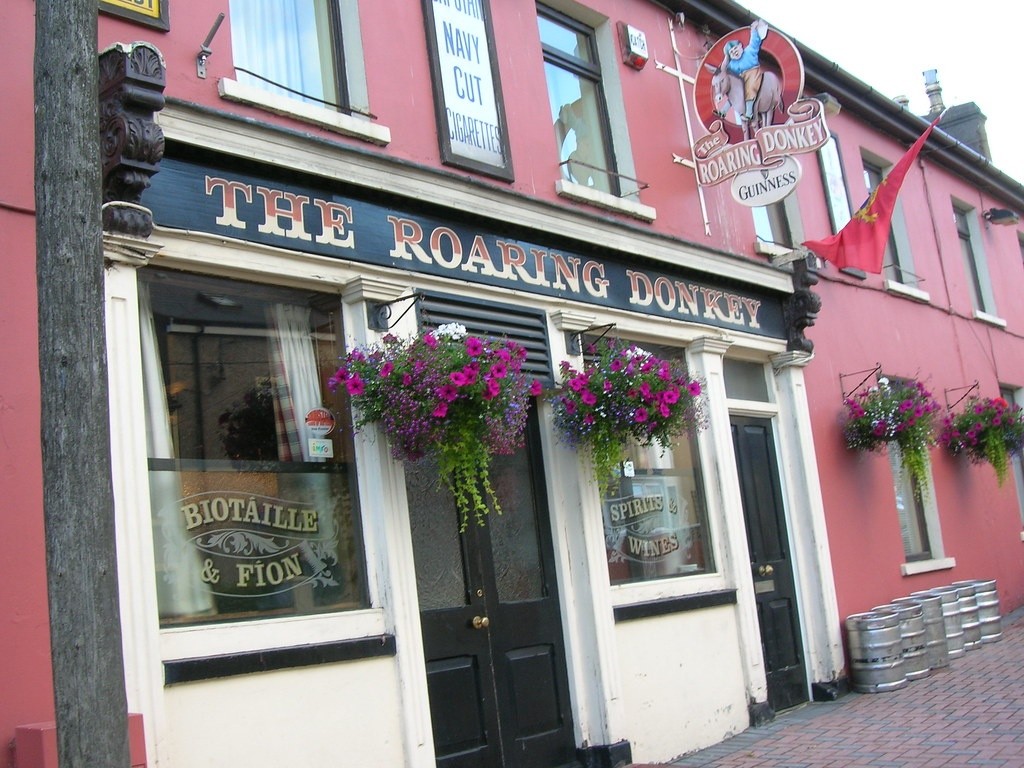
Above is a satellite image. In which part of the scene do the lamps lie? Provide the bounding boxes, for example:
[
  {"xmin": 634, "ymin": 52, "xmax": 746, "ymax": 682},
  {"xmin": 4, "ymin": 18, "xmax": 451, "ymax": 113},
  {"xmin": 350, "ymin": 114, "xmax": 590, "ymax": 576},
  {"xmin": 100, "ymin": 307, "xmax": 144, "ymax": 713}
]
[
  {"xmin": 984, "ymin": 207, "xmax": 1019, "ymax": 227},
  {"xmin": 812, "ymin": 92, "xmax": 843, "ymax": 120}
]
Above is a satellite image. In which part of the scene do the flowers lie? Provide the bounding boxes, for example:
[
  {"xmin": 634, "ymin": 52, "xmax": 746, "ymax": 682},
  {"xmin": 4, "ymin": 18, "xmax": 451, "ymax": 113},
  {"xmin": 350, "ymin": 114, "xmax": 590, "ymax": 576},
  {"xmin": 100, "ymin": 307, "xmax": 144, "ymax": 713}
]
[
  {"xmin": 840, "ymin": 365, "xmax": 947, "ymax": 499},
  {"xmin": 936, "ymin": 393, "xmax": 1024, "ymax": 488},
  {"xmin": 330, "ymin": 321, "xmax": 542, "ymax": 534},
  {"xmin": 542, "ymin": 330, "xmax": 712, "ymax": 506}
]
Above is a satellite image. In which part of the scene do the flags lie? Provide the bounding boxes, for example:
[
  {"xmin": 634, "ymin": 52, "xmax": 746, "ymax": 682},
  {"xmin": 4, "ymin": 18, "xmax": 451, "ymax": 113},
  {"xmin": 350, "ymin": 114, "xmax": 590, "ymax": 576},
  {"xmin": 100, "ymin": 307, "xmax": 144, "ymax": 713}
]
[{"xmin": 797, "ymin": 115, "xmax": 944, "ymax": 275}]
